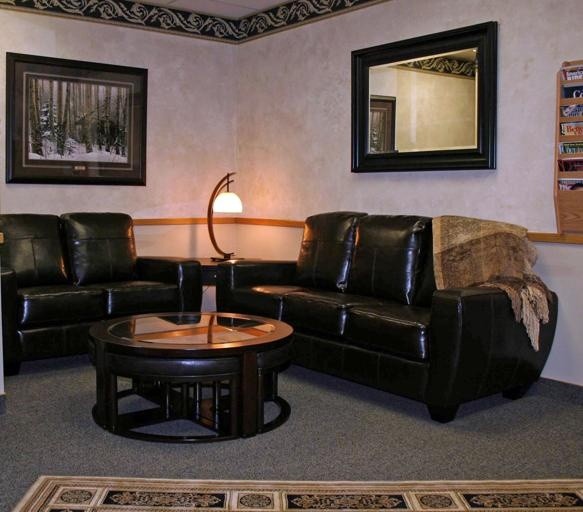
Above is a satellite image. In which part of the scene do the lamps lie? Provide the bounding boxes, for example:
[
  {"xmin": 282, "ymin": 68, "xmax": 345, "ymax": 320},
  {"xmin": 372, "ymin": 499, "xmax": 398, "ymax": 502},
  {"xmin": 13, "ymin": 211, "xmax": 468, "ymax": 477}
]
[{"xmin": 207, "ymin": 171, "xmax": 245, "ymax": 262}]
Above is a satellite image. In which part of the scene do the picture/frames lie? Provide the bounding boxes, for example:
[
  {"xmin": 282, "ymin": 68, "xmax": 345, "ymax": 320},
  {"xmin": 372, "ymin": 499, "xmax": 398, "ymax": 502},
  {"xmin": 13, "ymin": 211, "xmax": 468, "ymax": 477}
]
[
  {"xmin": 3, "ymin": 52, "xmax": 149, "ymax": 187},
  {"xmin": 369, "ymin": 94, "xmax": 398, "ymax": 154}
]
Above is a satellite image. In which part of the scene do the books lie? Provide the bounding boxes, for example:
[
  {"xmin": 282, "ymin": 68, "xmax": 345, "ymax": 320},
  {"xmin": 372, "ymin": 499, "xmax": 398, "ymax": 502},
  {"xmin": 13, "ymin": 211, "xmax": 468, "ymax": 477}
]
[{"xmin": 557, "ymin": 68, "xmax": 582, "ymax": 190}]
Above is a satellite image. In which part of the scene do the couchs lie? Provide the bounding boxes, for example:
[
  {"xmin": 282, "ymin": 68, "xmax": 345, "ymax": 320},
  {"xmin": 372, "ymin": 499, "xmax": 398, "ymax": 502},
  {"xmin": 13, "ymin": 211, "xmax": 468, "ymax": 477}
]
[
  {"xmin": 217, "ymin": 211, "xmax": 559, "ymax": 423},
  {"xmin": 0, "ymin": 213, "xmax": 204, "ymax": 377}
]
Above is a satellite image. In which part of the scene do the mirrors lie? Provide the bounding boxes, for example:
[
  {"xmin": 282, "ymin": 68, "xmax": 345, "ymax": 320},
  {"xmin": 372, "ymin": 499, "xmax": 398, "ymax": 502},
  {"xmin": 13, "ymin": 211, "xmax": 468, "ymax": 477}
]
[{"xmin": 351, "ymin": 19, "xmax": 499, "ymax": 173}]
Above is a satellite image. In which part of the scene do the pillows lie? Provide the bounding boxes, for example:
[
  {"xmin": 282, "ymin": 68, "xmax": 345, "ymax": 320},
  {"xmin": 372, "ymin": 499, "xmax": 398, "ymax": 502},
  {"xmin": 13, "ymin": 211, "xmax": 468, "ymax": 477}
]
[
  {"xmin": 294, "ymin": 212, "xmax": 369, "ymax": 293},
  {"xmin": 350, "ymin": 215, "xmax": 435, "ymax": 306},
  {"xmin": 61, "ymin": 213, "xmax": 139, "ymax": 287},
  {"xmin": 414, "ymin": 227, "xmax": 437, "ymax": 304},
  {"xmin": 0, "ymin": 214, "xmax": 68, "ymax": 288}
]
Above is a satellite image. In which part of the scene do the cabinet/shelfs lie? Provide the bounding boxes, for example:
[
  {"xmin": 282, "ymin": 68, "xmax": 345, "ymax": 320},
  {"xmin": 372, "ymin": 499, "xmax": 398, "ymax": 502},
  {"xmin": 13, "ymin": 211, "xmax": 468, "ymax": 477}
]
[{"xmin": 554, "ymin": 60, "xmax": 583, "ymax": 235}]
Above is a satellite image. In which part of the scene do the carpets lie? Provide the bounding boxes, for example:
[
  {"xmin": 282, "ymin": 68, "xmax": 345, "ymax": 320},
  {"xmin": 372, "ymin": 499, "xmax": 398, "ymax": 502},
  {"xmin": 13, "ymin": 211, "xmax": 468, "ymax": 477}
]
[{"xmin": 14, "ymin": 475, "xmax": 583, "ymax": 512}]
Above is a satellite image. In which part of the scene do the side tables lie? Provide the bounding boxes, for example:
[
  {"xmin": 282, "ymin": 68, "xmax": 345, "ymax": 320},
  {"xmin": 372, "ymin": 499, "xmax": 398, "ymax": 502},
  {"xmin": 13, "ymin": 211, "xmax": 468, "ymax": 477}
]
[{"xmin": 196, "ymin": 257, "xmax": 223, "ymax": 286}]
